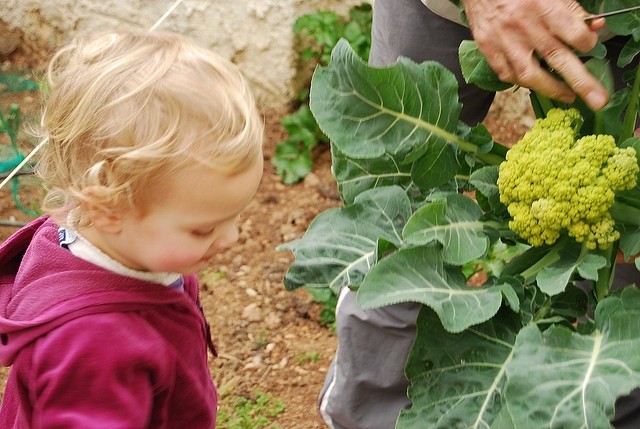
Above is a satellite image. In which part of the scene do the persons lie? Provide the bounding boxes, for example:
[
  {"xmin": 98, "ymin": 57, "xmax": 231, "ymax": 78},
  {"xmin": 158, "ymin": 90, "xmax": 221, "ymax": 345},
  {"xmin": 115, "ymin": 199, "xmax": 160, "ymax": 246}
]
[
  {"xmin": 0, "ymin": 31, "xmax": 264, "ymax": 429},
  {"xmin": 317, "ymin": 0, "xmax": 640, "ymax": 429}
]
